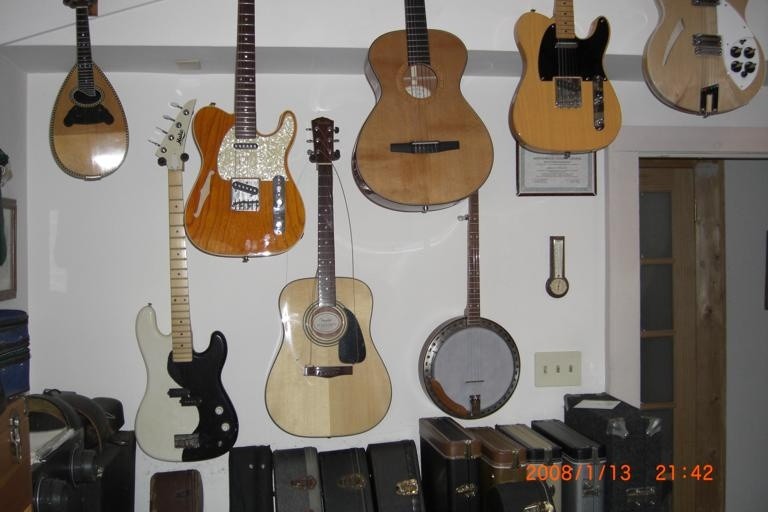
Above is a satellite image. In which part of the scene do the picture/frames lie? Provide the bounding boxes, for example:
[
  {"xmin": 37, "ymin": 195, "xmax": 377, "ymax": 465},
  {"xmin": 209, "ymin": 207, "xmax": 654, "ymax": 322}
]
[
  {"xmin": 514, "ymin": 138, "xmax": 599, "ymax": 198},
  {"xmin": 0, "ymin": 195, "xmax": 20, "ymax": 304}
]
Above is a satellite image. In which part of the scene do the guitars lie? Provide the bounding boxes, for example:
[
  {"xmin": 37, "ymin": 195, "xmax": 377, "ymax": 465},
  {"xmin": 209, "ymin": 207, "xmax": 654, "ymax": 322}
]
[
  {"xmin": 508, "ymin": 1, "xmax": 622, "ymax": 153},
  {"xmin": 640, "ymin": 0, "xmax": 764, "ymax": 114},
  {"xmin": 184, "ymin": 0, "xmax": 304, "ymax": 260},
  {"xmin": 351, "ymin": 0, "xmax": 494, "ymax": 213},
  {"xmin": 262, "ymin": 117, "xmax": 394, "ymax": 440},
  {"xmin": 134, "ymin": 98, "xmax": 239, "ymax": 462}
]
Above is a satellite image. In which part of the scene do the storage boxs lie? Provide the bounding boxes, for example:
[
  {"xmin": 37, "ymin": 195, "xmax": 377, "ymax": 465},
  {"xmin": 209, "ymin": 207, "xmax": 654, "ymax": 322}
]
[{"xmin": 0, "ymin": 398, "xmax": 34, "ymax": 512}]
